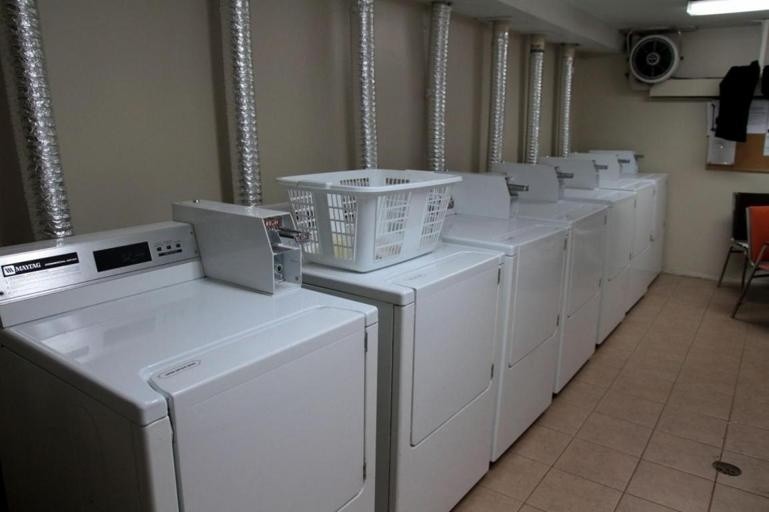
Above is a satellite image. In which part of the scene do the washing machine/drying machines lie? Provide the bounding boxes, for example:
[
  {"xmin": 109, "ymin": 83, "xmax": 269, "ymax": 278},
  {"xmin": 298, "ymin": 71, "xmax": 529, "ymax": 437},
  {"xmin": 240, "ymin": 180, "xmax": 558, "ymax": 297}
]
[{"xmin": 0, "ymin": 148, "xmax": 671, "ymax": 512}]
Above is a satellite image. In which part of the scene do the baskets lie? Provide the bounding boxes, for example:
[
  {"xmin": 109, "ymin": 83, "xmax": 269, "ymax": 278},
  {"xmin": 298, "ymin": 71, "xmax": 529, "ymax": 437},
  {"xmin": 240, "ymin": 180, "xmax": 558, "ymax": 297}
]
[{"xmin": 275, "ymin": 168, "xmax": 463, "ymax": 273}]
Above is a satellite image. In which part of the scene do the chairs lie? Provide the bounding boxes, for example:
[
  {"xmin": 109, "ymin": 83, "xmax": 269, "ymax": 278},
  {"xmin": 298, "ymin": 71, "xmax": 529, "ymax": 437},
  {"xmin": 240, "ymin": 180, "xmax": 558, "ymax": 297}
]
[{"xmin": 716, "ymin": 191, "xmax": 768, "ymax": 321}]
